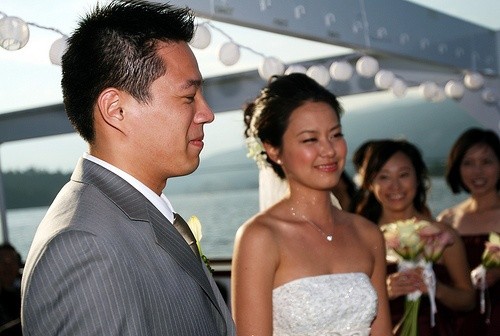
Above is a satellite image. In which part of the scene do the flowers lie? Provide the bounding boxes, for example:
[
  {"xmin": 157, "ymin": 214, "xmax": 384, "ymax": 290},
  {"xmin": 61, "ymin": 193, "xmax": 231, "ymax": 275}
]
[
  {"xmin": 247, "ymin": 133, "xmax": 265, "ymax": 162},
  {"xmin": 470, "ymin": 231, "xmax": 500, "ymax": 312},
  {"xmin": 189, "ymin": 216, "xmax": 215, "ymax": 275},
  {"xmin": 380, "ymin": 217, "xmax": 456, "ymax": 336}
]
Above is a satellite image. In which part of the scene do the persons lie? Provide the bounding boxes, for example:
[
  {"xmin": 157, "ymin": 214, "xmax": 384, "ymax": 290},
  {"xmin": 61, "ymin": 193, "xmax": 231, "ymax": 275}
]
[
  {"xmin": 0, "ymin": 240, "xmax": 28, "ymax": 336},
  {"xmin": 21, "ymin": 0, "xmax": 238, "ymax": 336},
  {"xmin": 230, "ymin": 74, "xmax": 393, "ymax": 336},
  {"xmin": 333, "ymin": 125, "xmax": 500, "ymax": 336}
]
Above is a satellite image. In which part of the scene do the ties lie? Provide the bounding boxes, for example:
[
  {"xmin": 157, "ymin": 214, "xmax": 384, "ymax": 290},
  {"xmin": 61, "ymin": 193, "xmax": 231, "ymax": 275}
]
[{"xmin": 171, "ymin": 211, "xmax": 203, "ymax": 265}]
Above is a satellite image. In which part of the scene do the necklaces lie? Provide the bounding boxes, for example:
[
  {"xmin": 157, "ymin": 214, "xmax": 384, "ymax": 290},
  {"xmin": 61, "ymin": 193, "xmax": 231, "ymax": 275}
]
[{"xmin": 293, "ymin": 205, "xmax": 334, "ymax": 242}]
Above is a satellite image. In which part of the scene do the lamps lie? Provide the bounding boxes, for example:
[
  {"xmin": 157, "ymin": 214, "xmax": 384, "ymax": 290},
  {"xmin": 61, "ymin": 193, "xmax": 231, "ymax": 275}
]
[
  {"xmin": 284, "ymin": 63, "xmax": 306, "ymax": 76},
  {"xmin": 305, "ymin": 63, "xmax": 331, "ymax": 88},
  {"xmin": 355, "ymin": 55, "xmax": 380, "ymax": 79},
  {"xmin": 442, "ymin": 79, "xmax": 466, "ymax": 100},
  {"xmin": 329, "ymin": 60, "xmax": 352, "ymax": 82},
  {"xmin": 0, "ymin": 15, "xmax": 30, "ymax": 51},
  {"xmin": 257, "ymin": 55, "xmax": 285, "ymax": 82},
  {"xmin": 48, "ymin": 34, "xmax": 71, "ymax": 65},
  {"xmin": 218, "ymin": 42, "xmax": 240, "ymax": 65},
  {"xmin": 188, "ymin": 24, "xmax": 212, "ymax": 49},
  {"xmin": 464, "ymin": 72, "xmax": 484, "ymax": 91},
  {"xmin": 373, "ymin": 69, "xmax": 395, "ymax": 90},
  {"xmin": 392, "ymin": 77, "xmax": 408, "ymax": 98},
  {"xmin": 419, "ymin": 81, "xmax": 438, "ymax": 101}
]
